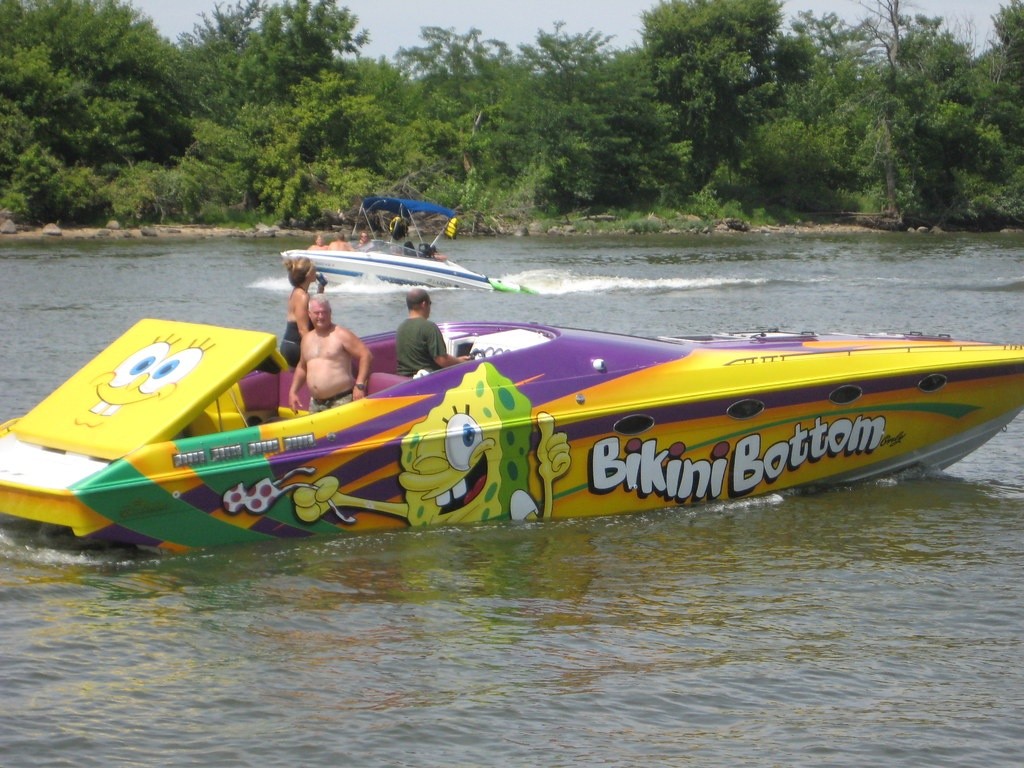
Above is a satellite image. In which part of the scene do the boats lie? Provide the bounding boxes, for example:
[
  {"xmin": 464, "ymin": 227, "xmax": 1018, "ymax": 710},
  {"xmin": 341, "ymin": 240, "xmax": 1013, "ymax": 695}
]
[
  {"xmin": 1, "ymin": 317, "xmax": 1023, "ymax": 561},
  {"xmin": 278, "ymin": 196, "xmax": 537, "ymax": 293}
]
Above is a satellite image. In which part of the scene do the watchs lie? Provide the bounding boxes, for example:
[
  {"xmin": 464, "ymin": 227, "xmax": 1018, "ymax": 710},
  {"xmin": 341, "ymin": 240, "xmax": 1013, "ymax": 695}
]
[{"xmin": 355, "ymin": 383, "xmax": 366, "ymax": 390}]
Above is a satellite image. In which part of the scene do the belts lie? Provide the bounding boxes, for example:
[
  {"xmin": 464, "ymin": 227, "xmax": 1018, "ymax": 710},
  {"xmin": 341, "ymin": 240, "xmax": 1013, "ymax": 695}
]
[{"xmin": 311, "ymin": 389, "xmax": 355, "ymax": 406}]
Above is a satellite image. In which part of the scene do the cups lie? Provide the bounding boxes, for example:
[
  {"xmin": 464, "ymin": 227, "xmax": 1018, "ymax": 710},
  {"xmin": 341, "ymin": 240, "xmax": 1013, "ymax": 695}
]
[{"xmin": 316, "ymin": 273, "xmax": 328, "ymax": 287}]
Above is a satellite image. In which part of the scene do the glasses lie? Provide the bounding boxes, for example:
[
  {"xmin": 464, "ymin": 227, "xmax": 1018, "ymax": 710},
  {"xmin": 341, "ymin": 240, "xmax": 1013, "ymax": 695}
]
[
  {"xmin": 424, "ymin": 300, "xmax": 432, "ymax": 305},
  {"xmin": 360, "ymin": 235, "xmax": 368, "ymax": 238}
]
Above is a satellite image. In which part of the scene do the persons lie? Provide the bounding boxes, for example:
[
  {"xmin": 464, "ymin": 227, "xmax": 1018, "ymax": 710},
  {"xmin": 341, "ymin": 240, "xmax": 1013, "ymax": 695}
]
[
  {"xmin": 325, "ymin": 234, "xmax": 355, "ymax": 250},
  {"xmin": 395, "ymin": 288, "xmax": 473, "ymax": 377},
  {"xmin": 287, "ymin": 293, "xmax": 372, "ymax": 415},
  {"xmin": 309, "ymin": 236, "xmax": 329, "ymax": 250},
  {"xmin": 280, "ymin": 253, "xmax": 328, "ymax": 366},
  {"xmin": 357, "ymin": 233, "xmax": 369, "ymax": 245}
]
